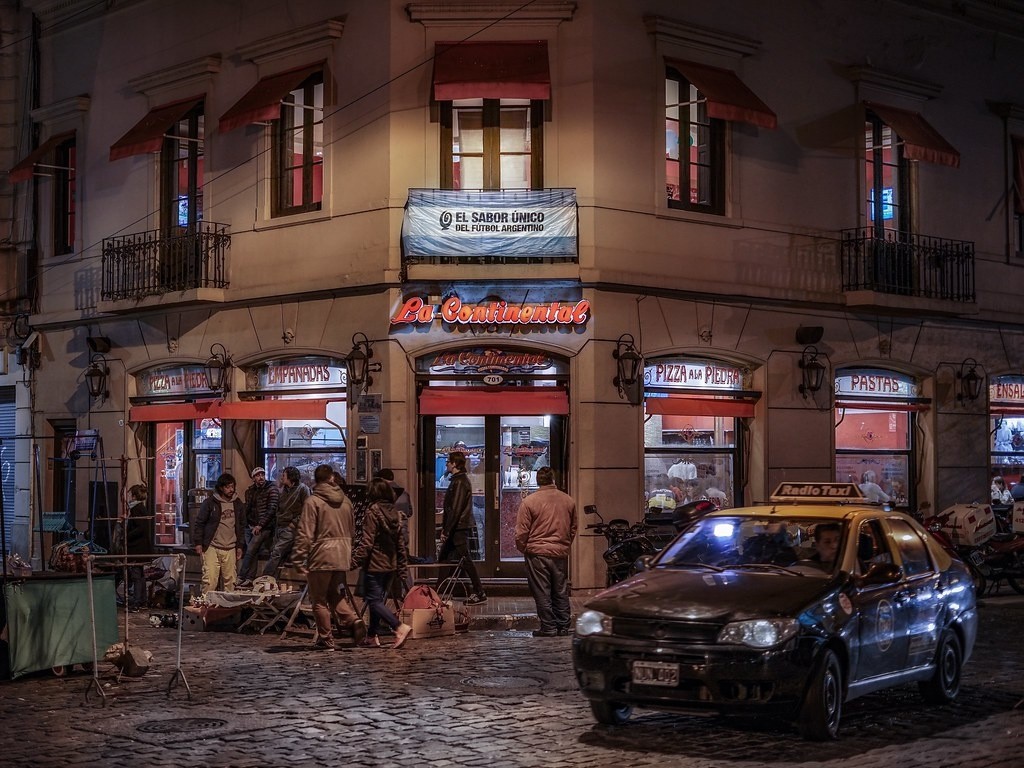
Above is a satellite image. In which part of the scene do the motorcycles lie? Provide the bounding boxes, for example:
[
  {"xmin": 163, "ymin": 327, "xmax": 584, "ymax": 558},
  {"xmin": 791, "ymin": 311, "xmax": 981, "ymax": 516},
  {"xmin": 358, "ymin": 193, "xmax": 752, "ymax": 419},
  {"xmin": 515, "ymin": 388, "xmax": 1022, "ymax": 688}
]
[
  {"xmin": 584, "ymin": 501, "xmax": 718, "ymax": 589},
  {"xmin": 887, "ymin": 506, "xmax": 1024, "ymax": 598}
]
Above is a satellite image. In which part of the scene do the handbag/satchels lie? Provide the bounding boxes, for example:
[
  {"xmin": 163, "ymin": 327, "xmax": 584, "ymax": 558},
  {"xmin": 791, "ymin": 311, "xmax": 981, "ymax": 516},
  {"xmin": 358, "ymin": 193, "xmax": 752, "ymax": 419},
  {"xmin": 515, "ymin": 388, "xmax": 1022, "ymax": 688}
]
[
  {"xmin": 403, "ymin": 584, "xmax": 443, "ymax": 608},
  {"xmin": 400, "ymin": 600, "xmax": 456, "ymax": 638}
]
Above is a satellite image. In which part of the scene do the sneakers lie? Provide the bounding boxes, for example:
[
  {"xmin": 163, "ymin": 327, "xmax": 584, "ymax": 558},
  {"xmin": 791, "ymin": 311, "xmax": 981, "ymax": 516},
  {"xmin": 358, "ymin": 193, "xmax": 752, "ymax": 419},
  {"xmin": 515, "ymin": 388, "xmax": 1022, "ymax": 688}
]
[
  {"xmin": 393, "ymin": 623, "xmax": 412, "ymax": 648},
  {"xmin": 463, "ymin": 592, "xmax": 488, "ymax": 605},
  {"xmin": 350, "ymin": 634, "xmax": 380, "ymax": 648}
]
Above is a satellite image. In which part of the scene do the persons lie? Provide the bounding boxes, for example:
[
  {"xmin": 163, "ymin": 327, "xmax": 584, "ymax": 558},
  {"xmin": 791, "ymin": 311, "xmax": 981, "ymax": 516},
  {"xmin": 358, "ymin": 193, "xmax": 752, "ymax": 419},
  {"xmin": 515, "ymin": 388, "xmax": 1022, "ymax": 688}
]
[
  {"xmin": 1010, "ymin": 476, "xmax": 1024, "ymax": 502},
  {"xmin": 990, "ymin": 477, "xmax": 1014, "ymax": 504},
  {"xmin": 888, "ymin": 480, "xmax": 908, "ymax": 505},
  {"xmin": 194, "ymin": 473, "xmax": 245, "ymax": 603},
  {"xmin": 437, "ymin": 452, "xmax": 488, "ymax": 606},
  {"xmin": 806, "ymin": 523, "xmax": 842, "ymax": 571},
  {"xmin": 646, "ymin": 474, "xmax": 729, "ymax": 514},
  {"xmin": 515, "ymin": 466, "xmax": 578, "ymax": 636},
  {"xmin": 291, "ymin": 465, "xmax": 367, "ymax": 652},
  {"xmin": 359, "ymin": 478, "xmax": 412, "ymax": 649},
  {"xmin": 235, "ymin": 467, "xmax": 279, "ymax": 590},
  {"xmin": 859, "ymin": 470, "xmax": 893, "ymax": 503},
  {"xmin": 122, "ymin": 485, "xmax": 153, "ymax": 612},
  {"xmin": 375, "ymin": 469, "xmax": 414, "ymax": 602},
  {"xmin": 262, "ymin": 467, "xmax": 312, "ymax": 579}
]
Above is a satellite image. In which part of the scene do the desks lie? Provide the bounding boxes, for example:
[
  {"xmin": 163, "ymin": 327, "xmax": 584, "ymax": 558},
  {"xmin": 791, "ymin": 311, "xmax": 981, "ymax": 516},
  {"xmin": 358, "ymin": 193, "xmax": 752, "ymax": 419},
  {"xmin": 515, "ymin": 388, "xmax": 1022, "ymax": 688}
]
[
  {"xmin": 206, "ymin": 560, "xmax": 465, "ymax": 640},
  {"xmin": 0, "ymin": 570, "xmax": 123, "ymax": 678}
]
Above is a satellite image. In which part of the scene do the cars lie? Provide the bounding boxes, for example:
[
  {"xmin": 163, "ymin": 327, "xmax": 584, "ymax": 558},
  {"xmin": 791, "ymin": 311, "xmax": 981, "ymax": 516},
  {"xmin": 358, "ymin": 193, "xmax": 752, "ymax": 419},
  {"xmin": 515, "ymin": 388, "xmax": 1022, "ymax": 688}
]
[{"xmin": 572, "ymin": 481, "xmax": 978, "ymax": 739}]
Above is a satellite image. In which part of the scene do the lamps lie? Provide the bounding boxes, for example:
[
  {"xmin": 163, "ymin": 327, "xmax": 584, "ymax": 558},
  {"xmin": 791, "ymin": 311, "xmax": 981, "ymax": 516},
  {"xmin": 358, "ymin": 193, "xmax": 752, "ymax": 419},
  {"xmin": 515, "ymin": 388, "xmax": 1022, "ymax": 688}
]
[
  {"xmin": 203, "ymin": 342, "xmax": 233, "ymax": 392},
  {"xmin": 612, "ymin": 332, "xmax": 646, "ymax": 391},
  {"xmin": 955, "ymin": 358, "xmax": 984, "ymax": 402},
  {"xmin": 84, "ymin": 356, "xmax": 111, "ymax": 400},
  {"xmin": 346, "ymin": 333, "xmax": 379, "ymax": 386},
  {"xmin": 799, "ymin": 345, "xmax": 826, "ymax": 396}
]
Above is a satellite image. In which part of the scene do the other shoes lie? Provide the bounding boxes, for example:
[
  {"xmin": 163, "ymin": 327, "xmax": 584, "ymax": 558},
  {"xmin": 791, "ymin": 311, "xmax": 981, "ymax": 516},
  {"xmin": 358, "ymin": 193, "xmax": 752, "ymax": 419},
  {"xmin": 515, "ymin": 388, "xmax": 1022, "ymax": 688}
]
[
  {"xmin": 131, "ymin": 604, "xmax": 149, "ymax": 612},
  {"xmin": 556, "ymin": 628, "xmax": 568, "ymax": 636},
  {"xmin": 532, "ymin": 629, "xmax": 557, "ymax": 637},
  {"xmin": 346, "ymin": 629, "xmax": 369, "ymax": 644},
  {"xmin": 302, "ymin": 633, "xmax": 334, "ymax": 652}
]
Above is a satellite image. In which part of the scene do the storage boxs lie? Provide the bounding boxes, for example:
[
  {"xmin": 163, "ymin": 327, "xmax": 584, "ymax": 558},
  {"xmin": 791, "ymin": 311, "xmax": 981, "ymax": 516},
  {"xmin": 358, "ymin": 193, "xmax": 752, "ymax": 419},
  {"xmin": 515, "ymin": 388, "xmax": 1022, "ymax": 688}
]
[{"xmin": 400, "ymin": 604, "xmax": 455, "ymax": 639}]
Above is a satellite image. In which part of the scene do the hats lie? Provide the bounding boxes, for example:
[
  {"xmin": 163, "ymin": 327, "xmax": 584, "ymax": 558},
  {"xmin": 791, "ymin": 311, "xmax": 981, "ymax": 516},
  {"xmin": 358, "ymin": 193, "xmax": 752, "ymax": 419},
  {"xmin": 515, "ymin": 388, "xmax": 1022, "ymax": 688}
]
[
  {"xmin": 376, "ymin": 468, "xmax": 395, "ymax": 483},
  {"xmin": 251, "ymin": 467, "xmax": 265, "ymax": 477}
]
